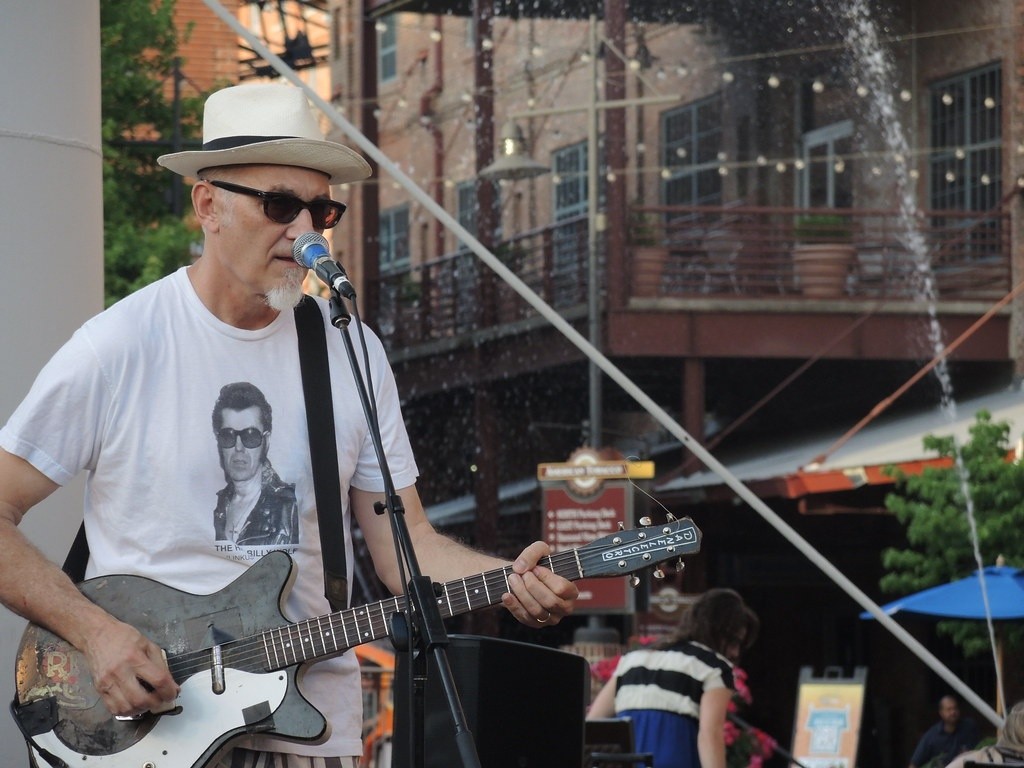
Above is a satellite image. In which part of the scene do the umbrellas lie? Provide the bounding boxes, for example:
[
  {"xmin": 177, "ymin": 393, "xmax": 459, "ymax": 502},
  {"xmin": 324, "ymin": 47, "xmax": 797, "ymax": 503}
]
[{"xmin": 859, "ymin": 555, "xmax": 1024, "ymax": 768}]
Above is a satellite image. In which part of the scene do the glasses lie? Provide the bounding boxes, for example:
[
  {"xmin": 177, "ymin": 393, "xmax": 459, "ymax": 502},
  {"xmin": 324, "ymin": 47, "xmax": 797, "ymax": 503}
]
[
  {"xmin": 216, "ymin": 426, "xmax": 271, "ymax": 449},
  {"xmin": 201, "ymin": 178, "xmax": 346, "ymax": 230},
  {"xmin": 725, "ymin": 634, "xmax": 743, "ymax": 649}
]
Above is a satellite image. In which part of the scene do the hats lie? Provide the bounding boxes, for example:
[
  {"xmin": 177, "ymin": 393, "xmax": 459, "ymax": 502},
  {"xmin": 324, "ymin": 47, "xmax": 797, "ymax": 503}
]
[{"xmin": 155, "ymin": 84, "xmax": 373, "ymax": 186}]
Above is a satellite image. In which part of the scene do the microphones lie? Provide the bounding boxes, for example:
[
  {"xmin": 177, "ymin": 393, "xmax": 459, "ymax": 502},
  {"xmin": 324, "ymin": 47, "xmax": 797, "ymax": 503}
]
[{"xmin": 292, "ymin": 232, "xmax": 357, "ymax": 300}]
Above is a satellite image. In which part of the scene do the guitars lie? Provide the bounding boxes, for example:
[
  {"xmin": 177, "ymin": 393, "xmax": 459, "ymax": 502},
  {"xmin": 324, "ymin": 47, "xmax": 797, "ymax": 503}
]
[{"xmin": 10, "ymin": 510, "xmax": 705, "ymax": 768}]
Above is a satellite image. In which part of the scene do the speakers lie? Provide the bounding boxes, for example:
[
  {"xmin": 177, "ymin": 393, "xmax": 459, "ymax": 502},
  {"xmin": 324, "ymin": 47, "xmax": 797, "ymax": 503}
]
[{"xmin": 391, "ymin": 634, "xmax": 589, "ymax": 768}]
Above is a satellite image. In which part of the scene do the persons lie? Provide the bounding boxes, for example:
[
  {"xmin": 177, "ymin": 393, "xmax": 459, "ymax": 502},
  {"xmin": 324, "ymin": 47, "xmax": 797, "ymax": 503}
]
[
  {"xmin": 0, "ymin": 79, "xmax": 579, "ymax": 768},
  {"xmin": 586, "ymin": 589, "xmax": 760, "ymax": 767},
  {"xmin": 944, "ymin": 701, "xmax": 1024, "ymax": 768},
  {"xmin": 909, "ymin": 694, "xmax": 966, "ymax": 768},
  {"xmin": 211, "ymin": 381, "xmax": 300, "ymax": 546}
]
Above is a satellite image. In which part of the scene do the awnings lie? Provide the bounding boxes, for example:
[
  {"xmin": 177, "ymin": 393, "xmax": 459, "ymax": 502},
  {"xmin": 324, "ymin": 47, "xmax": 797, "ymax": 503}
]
[{"xmin": 358, "ymin": 377, "xmax": 1023, "ymax": 538}]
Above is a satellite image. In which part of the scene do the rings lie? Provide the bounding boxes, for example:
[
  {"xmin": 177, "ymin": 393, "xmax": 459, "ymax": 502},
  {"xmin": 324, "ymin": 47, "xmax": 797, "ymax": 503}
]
[{"xmin": 537, "ymin": 612, "xmax": 552, "ymax": 623}]
